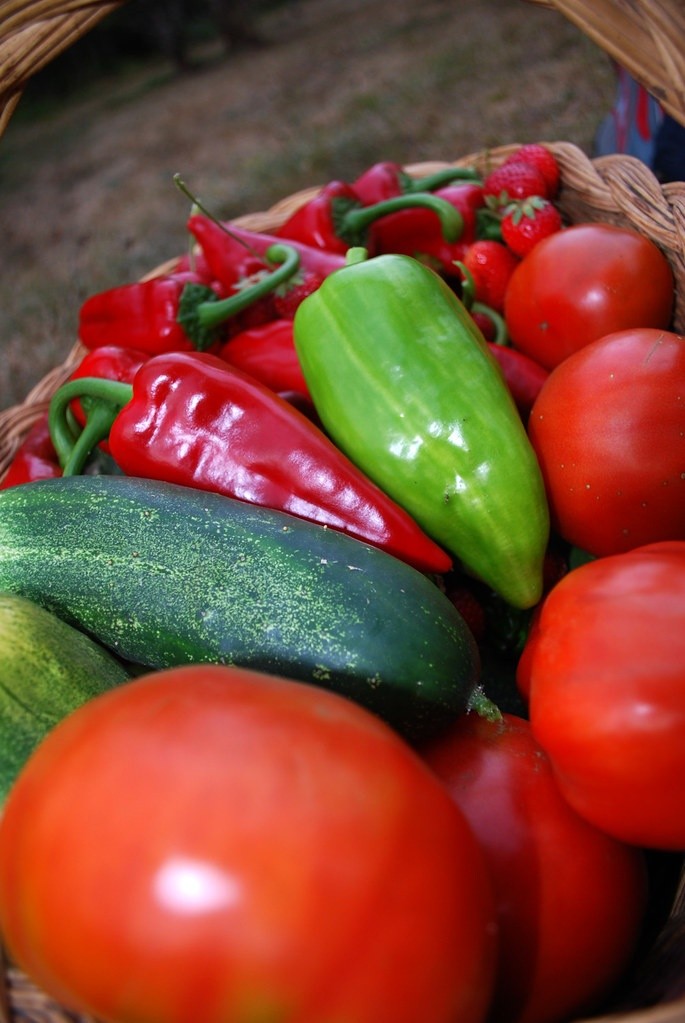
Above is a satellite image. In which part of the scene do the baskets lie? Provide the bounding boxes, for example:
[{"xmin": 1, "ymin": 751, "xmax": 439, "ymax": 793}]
[{"xmin": 3, "ymin": 2, "xmax": 683, "ymax": 1019}]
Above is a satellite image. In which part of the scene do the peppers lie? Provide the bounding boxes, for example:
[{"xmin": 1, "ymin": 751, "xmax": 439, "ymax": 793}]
[{"xmin": 0, "ymin": 162, "xmax": 569, "ymax": 627}]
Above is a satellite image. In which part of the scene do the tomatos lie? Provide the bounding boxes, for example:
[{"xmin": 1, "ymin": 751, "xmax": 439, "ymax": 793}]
[{"xmin": 1, "ymin": 223, "xmax": 684, "ymax": 1023}]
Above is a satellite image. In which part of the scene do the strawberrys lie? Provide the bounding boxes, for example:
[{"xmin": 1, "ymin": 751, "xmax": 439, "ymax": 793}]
[{"xmin": 451, "ymin": 142, "xmax": 562, "ymax": 307}]
